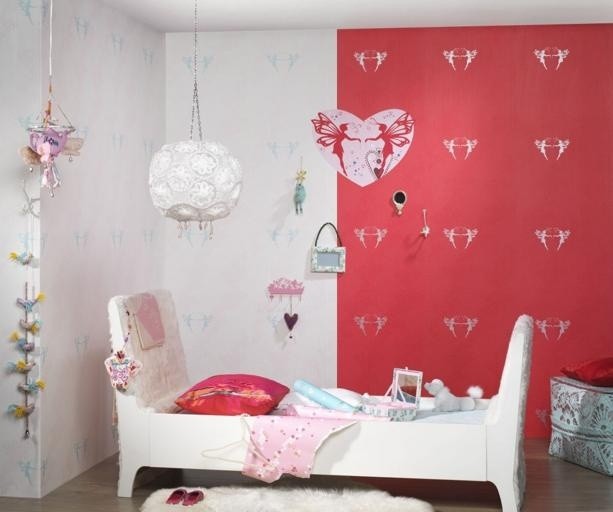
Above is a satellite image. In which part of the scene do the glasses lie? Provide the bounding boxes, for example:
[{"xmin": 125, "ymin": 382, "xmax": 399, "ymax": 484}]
[{"xmin": 142, "ymin": 0, "xmax": 244, "ymax": 240}]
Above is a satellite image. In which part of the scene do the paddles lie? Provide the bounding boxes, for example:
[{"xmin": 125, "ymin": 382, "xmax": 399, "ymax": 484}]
[{"xmin": 136, "ymin": 485, "xmax": 436, "ymax": 512}]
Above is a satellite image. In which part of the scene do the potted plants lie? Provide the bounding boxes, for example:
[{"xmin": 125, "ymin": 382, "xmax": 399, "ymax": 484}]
[{"xmin": 310, "ymin": 246, "xmax": 346, "ymax": 274}]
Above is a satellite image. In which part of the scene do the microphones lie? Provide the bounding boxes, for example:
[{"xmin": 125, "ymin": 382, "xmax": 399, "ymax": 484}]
[{"xmin": 545, "ymin": 374, "xmax": 612, "ymax": 477}]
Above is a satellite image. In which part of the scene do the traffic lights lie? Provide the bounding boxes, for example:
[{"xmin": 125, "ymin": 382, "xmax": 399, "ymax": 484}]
[
  {"xmin": 290, "ymin": 388, "xmax": 370, "ymax": 411},
  {"xmin": 557, "ymin": 354, "xmax": 611, "ymax": 390},
  {"xmin": 171, "ymin": 372, "xmax": 292, "ymax": 418}
]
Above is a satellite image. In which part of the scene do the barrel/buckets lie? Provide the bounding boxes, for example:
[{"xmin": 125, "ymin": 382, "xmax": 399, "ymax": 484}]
[
  {"xmin": 183, "ymin": 491, "xmax": 204, "ymax": 506},
  {"xmin": 166, "ymin": 489, "xmax": 187, "ymax": 504}
]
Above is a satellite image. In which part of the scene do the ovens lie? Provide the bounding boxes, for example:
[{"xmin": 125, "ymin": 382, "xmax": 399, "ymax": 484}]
[{"xmin": 101, "ymin": 283, "xmax": 536, "ymax": 512}]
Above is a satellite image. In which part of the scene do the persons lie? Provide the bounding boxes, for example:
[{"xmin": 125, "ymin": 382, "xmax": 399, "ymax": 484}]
[
  {"xmin": 332, "ymin": 123, "xmax": 361, "ymax": 176},
  {"xmin": 365, "ymin": 124, "xmax": 393, "ymax": 168}
]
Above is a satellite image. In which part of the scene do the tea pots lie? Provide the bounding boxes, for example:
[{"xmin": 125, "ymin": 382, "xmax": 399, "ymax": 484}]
[{"xmin": 390, "ymin": 368, "xmax": 423, "ymax": 410}]
